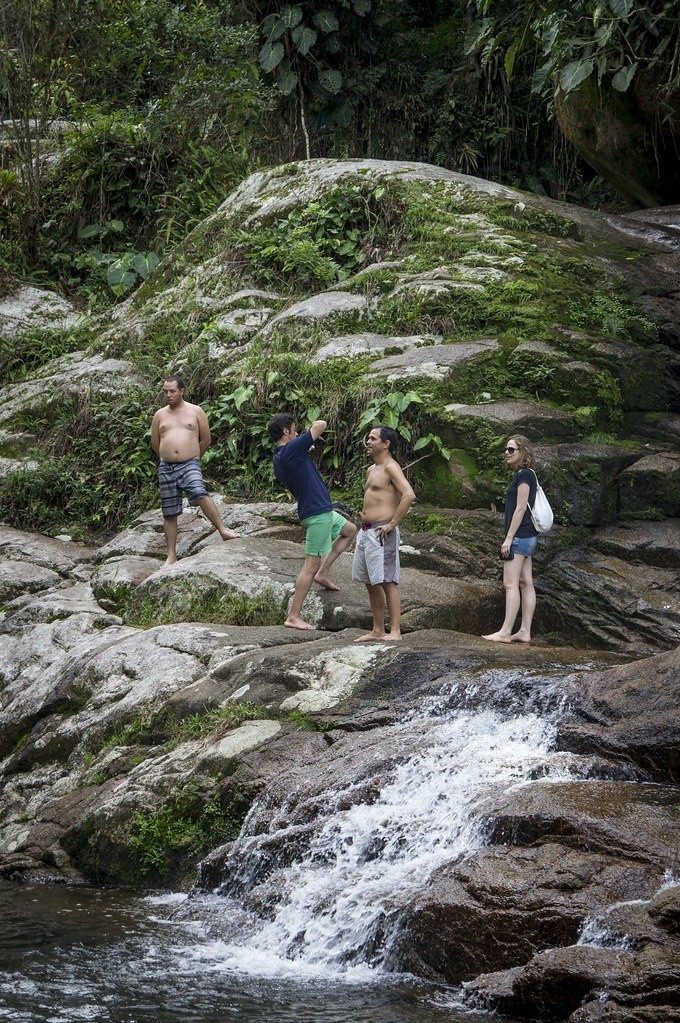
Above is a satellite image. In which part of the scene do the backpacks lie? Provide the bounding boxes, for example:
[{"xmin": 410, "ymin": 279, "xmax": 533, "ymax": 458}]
[{"xmin": 526, "ymin": 467, "xmax": 554, "ymax": 534}]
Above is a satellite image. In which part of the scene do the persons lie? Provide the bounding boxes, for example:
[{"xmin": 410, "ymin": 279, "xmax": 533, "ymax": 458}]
[
  {"xmin": 151, "ymin": 377, "xmax": 242, "ymax": 569},
  {"xmin": 481, "ymin": 434, "xmax": 540, "ymax": 644},
  {"xmin": 268, "ymin": 413, "xmax": 357, "ymax": 629},
  {"xmin": 351, "ymin": 425, "xmax": 416, "ymax": 642}
]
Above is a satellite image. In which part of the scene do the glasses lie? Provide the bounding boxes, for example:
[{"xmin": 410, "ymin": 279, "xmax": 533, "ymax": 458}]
[{"xmin": 505, "ymin": 447, "xmax": 520, "ymax": 454}]
